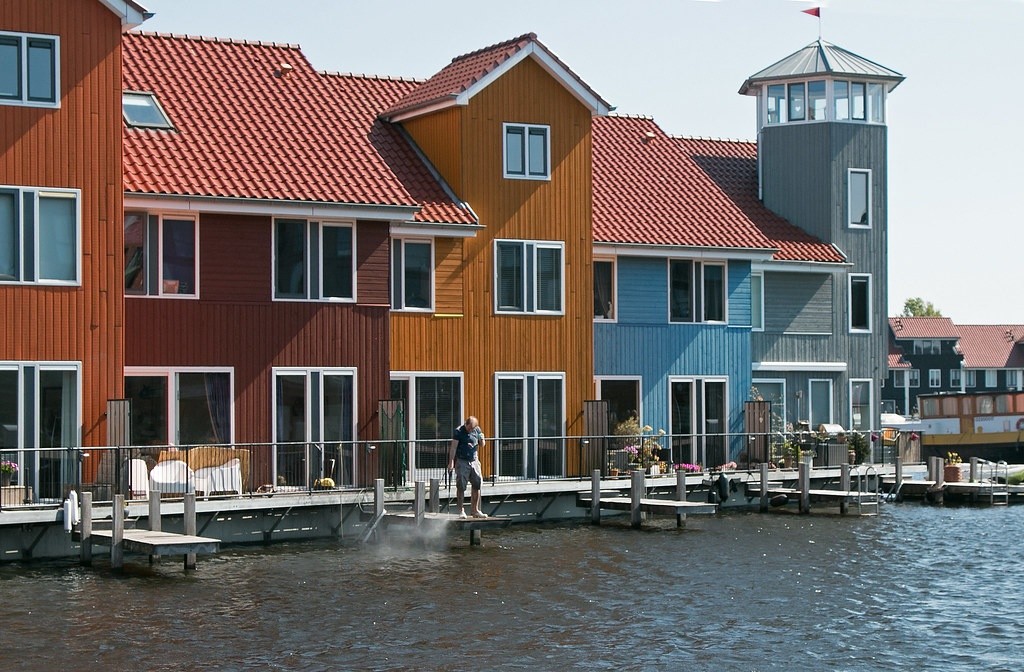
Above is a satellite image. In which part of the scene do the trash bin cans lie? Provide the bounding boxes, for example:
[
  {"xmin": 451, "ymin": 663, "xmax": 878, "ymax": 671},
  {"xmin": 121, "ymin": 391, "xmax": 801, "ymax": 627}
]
[{"xmin": 650, "ymin": 448, "xmax": 671, "ymax": 473}]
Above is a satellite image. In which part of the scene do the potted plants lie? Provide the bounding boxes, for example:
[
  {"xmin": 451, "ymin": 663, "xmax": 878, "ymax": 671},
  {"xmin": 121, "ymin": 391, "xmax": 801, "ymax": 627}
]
[
  {"xmin": 618, "ymin": 466, "xmax": 626, "ymax": 480},
  {"xmin": 770, "ymin": 428, "xmax": 870, "ymax": 468}
]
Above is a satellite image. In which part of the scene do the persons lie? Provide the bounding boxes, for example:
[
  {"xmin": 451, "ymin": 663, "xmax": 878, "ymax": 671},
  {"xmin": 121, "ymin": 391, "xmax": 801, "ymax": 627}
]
[{"xmin": 453, "ymin": 416, "xmax": 489, "ymax": 519}]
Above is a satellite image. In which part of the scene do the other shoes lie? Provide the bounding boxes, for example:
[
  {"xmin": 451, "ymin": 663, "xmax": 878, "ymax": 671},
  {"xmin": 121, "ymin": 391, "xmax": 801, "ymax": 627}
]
[
  {"xmin": 473, "ymin": 511, "xmax": 487, "ymax": 517},
  {"xmin": 459, "ymin": 511, "xmax": 467, "ymax": 519}
]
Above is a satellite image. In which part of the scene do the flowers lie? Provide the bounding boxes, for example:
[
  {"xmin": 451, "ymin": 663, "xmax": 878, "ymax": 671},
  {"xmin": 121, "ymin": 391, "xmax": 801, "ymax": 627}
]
[
  {"xmin": 944, "ymin": 452, "xmax": 962, "ymax": 465},
  {"xmin": 1, "ymin": 460, "xmax": 18, "ymax": 474},
  {"xmin": 610, "ymin": 416, "xmax": 668, "ymax": 465},
  {"xmin": 718, "ymin": 461, "xmax": 737, "ymax": 470},
  {"xmin": 674, "ymin": 463, "xmax": 700, "ymax": 472}
]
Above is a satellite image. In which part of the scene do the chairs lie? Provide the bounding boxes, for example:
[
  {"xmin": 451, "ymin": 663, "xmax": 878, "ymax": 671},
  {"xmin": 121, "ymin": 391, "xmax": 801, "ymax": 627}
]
[
  {"xmin": 194, "ymin": 458, "xmax": 242, "ymax": 501},
  {"xmin": 149, "ymin": 460, "xmax": 194, "ymax": 493},
  {"xmin": 120, "ymin": 459, "xmax": 149, "ymax": 499}
]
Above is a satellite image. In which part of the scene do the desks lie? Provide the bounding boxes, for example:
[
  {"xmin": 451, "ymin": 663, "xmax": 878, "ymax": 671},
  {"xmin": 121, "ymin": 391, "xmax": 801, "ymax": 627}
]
[{"xmin": 1, "ymin": 486, "xmax": 32, "ymax": 506}]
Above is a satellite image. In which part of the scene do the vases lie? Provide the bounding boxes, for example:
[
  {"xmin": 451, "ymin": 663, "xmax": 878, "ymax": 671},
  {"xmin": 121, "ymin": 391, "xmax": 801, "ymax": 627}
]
[
  {"xmin": 610, "ymin": 468, "xmax": 619, "ymax": 479},
  {"xmin": 944, "ymin": 465, "xmax": 962, "ymax": 482},
  {"xmin": 2, "ymin": 473, "xmax": 10, "ymax": 485},
  {"xmin": 628, "ymin": 463, "xmax": 642, "ymax": 475}
]
